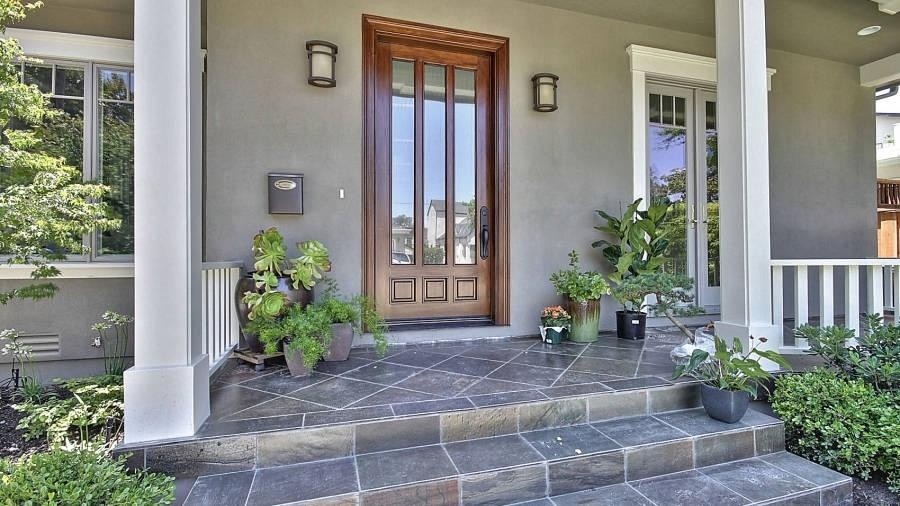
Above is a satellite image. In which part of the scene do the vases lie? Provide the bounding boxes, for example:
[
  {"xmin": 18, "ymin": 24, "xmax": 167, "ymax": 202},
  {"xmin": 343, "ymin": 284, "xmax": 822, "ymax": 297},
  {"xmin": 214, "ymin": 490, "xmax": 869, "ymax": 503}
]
[{"xmin": 540, "ymin": 317, "xmax": 566, "ymax": 345}]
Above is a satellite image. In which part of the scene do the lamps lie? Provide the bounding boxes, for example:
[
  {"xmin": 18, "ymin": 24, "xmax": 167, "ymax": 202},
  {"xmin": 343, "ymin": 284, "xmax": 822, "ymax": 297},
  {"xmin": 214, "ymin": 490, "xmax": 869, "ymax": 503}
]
[
  {"xmin": 531, "ymin": 73, "xmax": 559, "ymax": 112},
  {"xmin": 305, "ymin": 41, "xmax": 338, "ymax": 88}
]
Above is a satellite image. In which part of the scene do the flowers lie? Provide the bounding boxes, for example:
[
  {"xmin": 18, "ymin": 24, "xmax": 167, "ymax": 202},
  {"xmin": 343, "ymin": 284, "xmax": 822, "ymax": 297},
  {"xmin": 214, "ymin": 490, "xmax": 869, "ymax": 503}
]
[{"xmin": 541, "ymin": 305, "xmax": 572, "ymax": 319}]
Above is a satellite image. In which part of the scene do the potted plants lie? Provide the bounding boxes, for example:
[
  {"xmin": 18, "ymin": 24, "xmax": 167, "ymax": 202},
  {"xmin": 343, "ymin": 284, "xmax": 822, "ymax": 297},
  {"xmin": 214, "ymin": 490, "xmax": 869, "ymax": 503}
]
[
  {"xmin": 240, "ymin": 302, "xmax": 333, "ymax": 376},
  {"xmin": 591, "ymin": 198, "xmax": 706, "ymax": 340},
  {"xmin": 548, "ymin": 250, "xmax": 612, "ymax": 342},
  {"xmin": 318, "ymin": 276, "xmax": 390, "ymax": 362},
  {"xmin": 234, "ymin": 228, "xmax": 331, "ymax": 357},
  {"xmin": 668, "ymin": 335, "xmax": 793, "ymax": 424}
]
